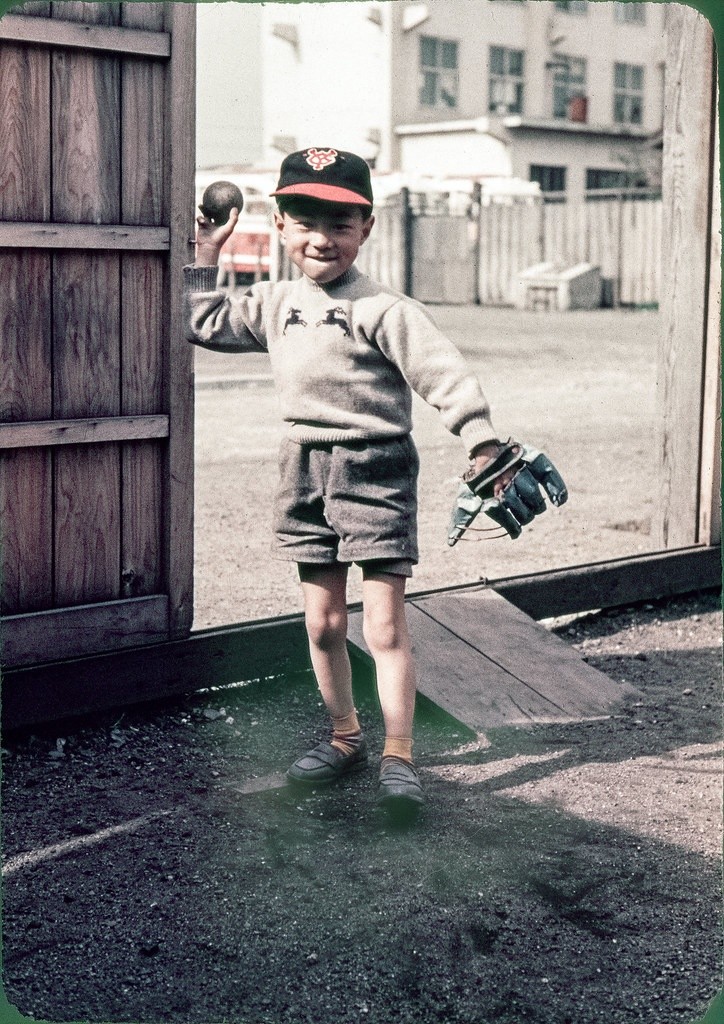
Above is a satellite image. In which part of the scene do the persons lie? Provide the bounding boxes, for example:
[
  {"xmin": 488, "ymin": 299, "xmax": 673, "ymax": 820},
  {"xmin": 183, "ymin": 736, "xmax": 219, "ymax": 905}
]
[{"xmin": 179, "ymin": 147, "xmax": 530, "ymax": 806}]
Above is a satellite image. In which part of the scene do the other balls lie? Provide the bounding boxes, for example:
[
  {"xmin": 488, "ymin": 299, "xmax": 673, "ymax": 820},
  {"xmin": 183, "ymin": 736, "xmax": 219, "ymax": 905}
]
[{"xmin": 202, "ymin": 180, "xmax": 244, "ymax": 223}]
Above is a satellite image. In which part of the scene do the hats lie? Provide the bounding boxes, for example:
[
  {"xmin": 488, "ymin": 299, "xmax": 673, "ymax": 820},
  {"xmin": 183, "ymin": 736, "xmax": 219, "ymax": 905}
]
[{"xmin": 269, "ymin": 147, "xmax": 373, "ymax": 217}]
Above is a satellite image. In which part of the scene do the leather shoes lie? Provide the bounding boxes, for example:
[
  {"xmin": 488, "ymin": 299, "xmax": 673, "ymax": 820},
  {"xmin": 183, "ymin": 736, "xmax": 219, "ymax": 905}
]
[
  {"xmin": 287, "ymin": 740, "xmax": 370, "ymax": 786},
  {"xmin": 374, "ymin": 757, "xmax": 425, "ymax": 808}
]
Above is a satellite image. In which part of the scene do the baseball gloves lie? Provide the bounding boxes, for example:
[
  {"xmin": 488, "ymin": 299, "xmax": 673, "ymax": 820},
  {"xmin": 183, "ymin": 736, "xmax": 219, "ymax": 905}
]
[{"xmin": 445, "ymin": 438, "xmax": 569, "ymax": 547}]
